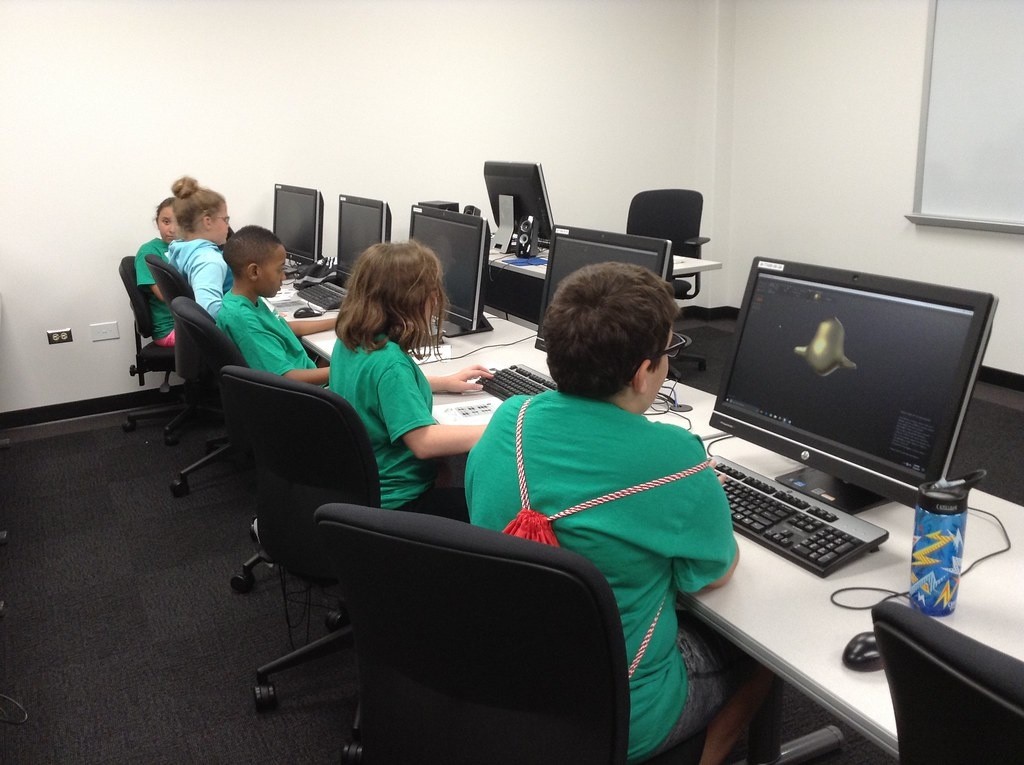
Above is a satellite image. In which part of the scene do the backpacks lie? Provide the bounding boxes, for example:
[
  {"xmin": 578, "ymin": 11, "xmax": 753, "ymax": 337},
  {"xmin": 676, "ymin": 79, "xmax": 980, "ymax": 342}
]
[{"xmin": 501, "ymin": 399, "xmax": 710, "ymax": 681}]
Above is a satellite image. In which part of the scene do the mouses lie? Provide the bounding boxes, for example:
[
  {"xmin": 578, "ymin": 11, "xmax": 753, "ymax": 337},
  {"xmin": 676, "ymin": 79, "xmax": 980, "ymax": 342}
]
[
  {"xmin": 843, "ymin": 632, "xmax": 882, "ymax": 671},
  {"xmin": 294, "ymin": 307, "xmax": 322, "ymax": 318}
]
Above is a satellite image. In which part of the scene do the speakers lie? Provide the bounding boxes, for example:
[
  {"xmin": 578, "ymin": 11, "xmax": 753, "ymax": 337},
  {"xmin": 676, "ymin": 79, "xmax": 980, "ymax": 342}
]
[
  {"xmin": 464, "ymin": 205, "xmax": 481, "ymax": 218},
  {"xmin": 515, "ymin": 215, "xmax": 539, "ymax": 258}
]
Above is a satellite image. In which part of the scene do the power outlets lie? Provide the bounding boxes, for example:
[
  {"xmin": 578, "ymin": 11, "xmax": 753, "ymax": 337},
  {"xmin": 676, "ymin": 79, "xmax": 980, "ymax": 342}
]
[
  {"xmin": 90, "ymin": 322, "xmax": 119, "ymax": 342},
  {"xmin": 47, "ymin": 327, "xmax": 73, "ymax": 344}
]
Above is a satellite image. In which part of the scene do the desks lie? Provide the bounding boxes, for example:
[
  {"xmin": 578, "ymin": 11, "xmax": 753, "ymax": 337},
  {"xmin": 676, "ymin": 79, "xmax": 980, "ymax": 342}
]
[
  {"xmin": 677, "ymin": 435, "xmax": 1024, "ymax": 762},
  {"xmin": 278, "ymin": 304, "xmax": 726, "ymax": 439},
  {"xmin": 488, "ymin": 233, "xmax": 723, "ymax": 283}
]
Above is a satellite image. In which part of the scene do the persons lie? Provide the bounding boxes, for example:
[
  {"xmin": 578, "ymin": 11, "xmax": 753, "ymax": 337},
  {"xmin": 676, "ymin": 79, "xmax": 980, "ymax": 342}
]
[
  {"xmin": 465, "ymin": 262, "xmax": 775, "ymax": 765},
  {"xmin": 328, "ymin": 244, "xmax": 494, "ymax": 524},
  {"xmin": 216, "ymin": 225, "xmax": 337, "ymax": 389},
  {"xmin": 135, "ymin": 198, "xmax": 179, "ymax": 346},
  {"xmin": 168, "ymin": 176, "xmax": 287, "ymax": 319}
]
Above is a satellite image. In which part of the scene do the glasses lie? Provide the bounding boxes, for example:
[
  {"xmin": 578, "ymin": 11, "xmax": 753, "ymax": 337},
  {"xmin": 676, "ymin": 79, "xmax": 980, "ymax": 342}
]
[
  {"xmin": 202, "ymin": 215, "xmax": 230, "ymax": 224},
  {"xmin": 627, "ymin": 331, "xmax": 686, "ymax": 382}
]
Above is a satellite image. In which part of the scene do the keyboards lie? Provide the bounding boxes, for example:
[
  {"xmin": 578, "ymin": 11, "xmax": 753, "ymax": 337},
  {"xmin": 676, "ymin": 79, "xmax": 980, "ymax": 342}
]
[
  {"xmin": 707, "ymin": 453, "xmax": 890, "ymax": 580},
  {"xmin": 297, "ymin": 282, "xmax": 349, "ymax": 310},
  {"xmin": 537, "ymin": 236, "xmax": 552, "ymax": 250},
  {"xmin": 475, "ymin": 364, "xmax": 558, "ymax": 401}
]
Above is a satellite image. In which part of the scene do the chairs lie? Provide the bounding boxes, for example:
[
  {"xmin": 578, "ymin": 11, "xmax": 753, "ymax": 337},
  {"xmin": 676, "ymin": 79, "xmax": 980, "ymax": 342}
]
[
  {"xmin": 870, "ymin": 601, "xmax": 1024, "ymax": 765},
  {"xmin": 625, "ymin": 189, "xmax": 710, "ymax": 380},
  {"xmin": 118, "ymin": 255, "xmax": 707, "ymax": 765}
]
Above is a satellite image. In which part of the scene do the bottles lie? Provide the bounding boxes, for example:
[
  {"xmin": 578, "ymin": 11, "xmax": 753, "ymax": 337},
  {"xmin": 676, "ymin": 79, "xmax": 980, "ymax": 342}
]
[{"xmin": 911, "ymin": 467, "xmax": 988, "ymax": 615}]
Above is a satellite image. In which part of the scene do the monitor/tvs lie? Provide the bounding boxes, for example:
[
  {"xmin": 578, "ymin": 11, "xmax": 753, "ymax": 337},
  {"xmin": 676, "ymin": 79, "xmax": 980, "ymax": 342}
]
[
  {"xmin": 709, "ymin": 256, "xmax": 999, "ymax": 513},
  {"xmin": 276, "ymin": 161, "xmax": 672, "ymax": 352}
]
